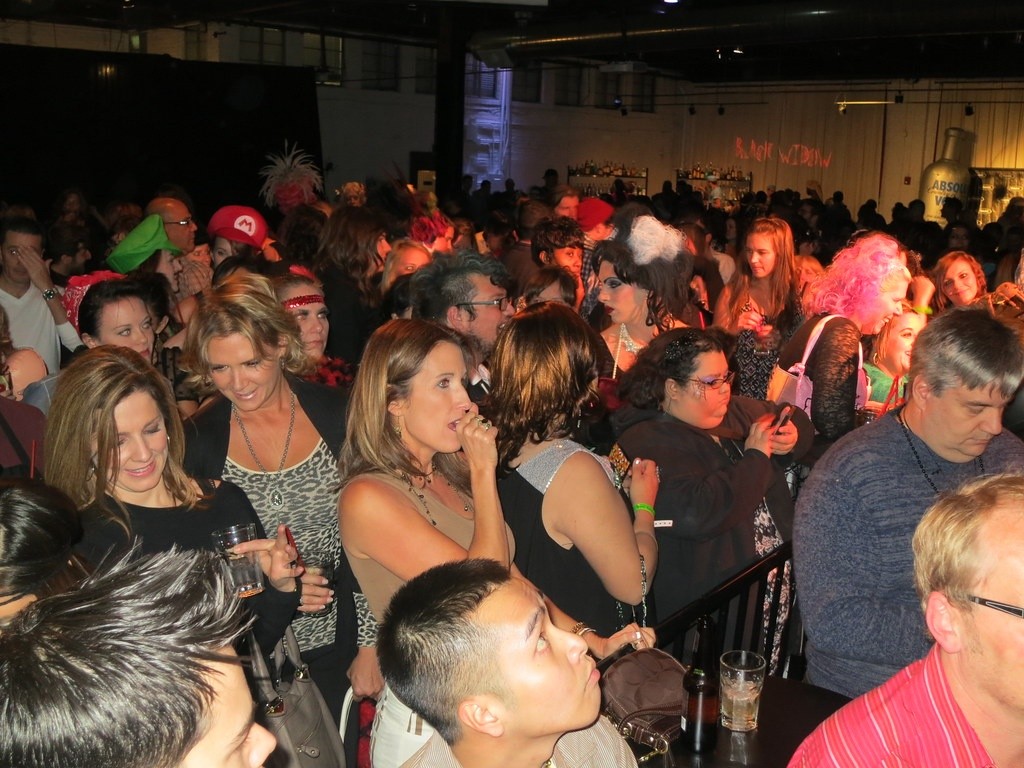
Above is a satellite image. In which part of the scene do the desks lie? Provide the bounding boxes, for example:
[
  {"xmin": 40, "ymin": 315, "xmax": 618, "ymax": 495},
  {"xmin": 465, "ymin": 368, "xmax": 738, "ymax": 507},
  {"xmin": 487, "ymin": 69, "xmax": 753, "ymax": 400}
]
[{"xmin": 624, "ymin": 667, "xmax": 854, "ymax": 768}]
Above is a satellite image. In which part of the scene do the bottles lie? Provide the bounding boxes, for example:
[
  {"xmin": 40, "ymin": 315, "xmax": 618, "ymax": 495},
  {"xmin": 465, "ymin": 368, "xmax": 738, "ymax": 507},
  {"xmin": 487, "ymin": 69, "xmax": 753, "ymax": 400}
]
[{"xmin": 680, "ymin": 614, "xmax": 720, "ymax": 754}]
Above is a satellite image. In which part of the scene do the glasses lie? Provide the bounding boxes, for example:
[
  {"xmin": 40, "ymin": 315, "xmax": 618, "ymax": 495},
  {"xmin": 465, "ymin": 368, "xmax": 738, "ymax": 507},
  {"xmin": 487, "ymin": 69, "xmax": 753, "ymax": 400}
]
[
  {"xmin": 671, "ymin": 370, "xmax": 735, "ymax": 389},
  {"xmin": 456, "ymin": 296, "xmax": 513, "ymax": 311},
  {"xmin": 164, "ymin": 217, "xmax": 192, "ymax": 225}
]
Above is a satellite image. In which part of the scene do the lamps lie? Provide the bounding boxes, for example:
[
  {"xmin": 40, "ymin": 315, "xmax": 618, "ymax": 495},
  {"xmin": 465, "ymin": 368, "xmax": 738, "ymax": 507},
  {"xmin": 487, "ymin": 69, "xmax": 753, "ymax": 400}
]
[
  {"xmin": 894, "ymin": 90, "xmax": 904, "ymax": 104},
  {"xmin": 688, "ymin": 104, "xmax": 696, "ymax": 114},
  {"xmin": 620, "ymin": 106, "xmax": 628, "ymax": 117},
  {"xmin": 734, "ymin": 43, "xmax": 744, "ymax": 53},
  {"xmin": 717, "ymin": 103, "xmax": 725, "ymax": 115},
  {"xmin": 839, "ymin": 104, "xmax": 847, "ymax": 115},
  {"xmin": 965, "ymin": 105, "xmax": 974, "ymax": 116}
]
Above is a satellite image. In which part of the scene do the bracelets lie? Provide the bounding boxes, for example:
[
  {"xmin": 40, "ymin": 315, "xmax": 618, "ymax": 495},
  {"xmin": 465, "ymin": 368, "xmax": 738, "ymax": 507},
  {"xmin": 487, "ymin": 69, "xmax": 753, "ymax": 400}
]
[
  {"xmin": 633, "ymin": 503, "xmax": 655, "ymax": 517},
  {"xmin": 570, "ymin": 621, "xmax": 590, "ymax": 636}
]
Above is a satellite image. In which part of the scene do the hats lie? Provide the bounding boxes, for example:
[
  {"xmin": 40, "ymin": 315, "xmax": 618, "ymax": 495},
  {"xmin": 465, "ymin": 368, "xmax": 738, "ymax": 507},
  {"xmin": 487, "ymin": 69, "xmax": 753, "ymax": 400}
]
[
  {"xmin": 576, "ymin": 198, "xmax": 614, "ymax": 231},
  {"xmin": 206, "ymin": 205, "xmax": 269, "ymax": 251}
]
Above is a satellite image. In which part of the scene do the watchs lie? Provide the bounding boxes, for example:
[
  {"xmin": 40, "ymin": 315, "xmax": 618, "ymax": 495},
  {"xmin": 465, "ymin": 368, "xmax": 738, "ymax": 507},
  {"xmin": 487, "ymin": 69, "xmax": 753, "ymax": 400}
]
[{"xmin": 43, "ymin": 288, "xmax": 57, "ymax": 301}]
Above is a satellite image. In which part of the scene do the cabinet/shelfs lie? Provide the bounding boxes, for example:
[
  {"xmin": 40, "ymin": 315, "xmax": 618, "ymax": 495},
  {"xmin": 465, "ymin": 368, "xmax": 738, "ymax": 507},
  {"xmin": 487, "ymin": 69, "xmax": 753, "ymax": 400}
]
[
  {"xmin": 567, "ymin": 165, "xmax": 648, "ymax": 196},
  {"xmin": 676, "ymin": 169, "xmax": 752, "ymax": 204}
]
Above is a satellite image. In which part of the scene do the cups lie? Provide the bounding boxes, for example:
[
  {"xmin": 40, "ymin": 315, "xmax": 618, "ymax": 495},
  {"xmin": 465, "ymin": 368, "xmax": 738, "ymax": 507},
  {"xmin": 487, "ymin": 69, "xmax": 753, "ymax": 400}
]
[
  {"xmin": 753, "ymin": 324, "xmax": 770, "ymax": 358},
  {"xmin": 211, "ymin": 523, "xmax": 265, "ymax": 597},
  {"xmin": 718, "ymin": 651, "xmax": 765, "ymax": 731},
  {"xmin": 862, "ymin": 406, "xmax": 882, "ymax": 425}
]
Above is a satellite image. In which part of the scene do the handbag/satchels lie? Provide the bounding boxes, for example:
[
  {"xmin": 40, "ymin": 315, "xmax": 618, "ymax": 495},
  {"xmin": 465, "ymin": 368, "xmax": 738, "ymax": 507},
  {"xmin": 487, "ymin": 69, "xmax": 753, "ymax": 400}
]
[
  {"xmin": 766, "ymin": 314, "xmax": 868, "ymax": 436},
  {"xmin": 601, "ymin": 647, "xmax": 690, "ymax": 768},
  {"xmin": 248, "ymin": 625, "xmax": 346, "ymax": 768}
]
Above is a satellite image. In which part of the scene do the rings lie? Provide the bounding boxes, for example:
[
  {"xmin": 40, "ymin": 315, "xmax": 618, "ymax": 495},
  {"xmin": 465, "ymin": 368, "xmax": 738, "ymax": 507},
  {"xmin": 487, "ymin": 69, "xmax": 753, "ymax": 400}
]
[
  {"xmin": 300, "ymin": 597, "xmax": 303, "ymax": 605},
  {"xmin": 475, "ymin": 417, "xmax": 479, "ymax": 420},
  {"xmin": 635, "ymin": 640, "xmax": 643, "ymax": 646},
  {"xmin": 479, "ymin": 419, "xmax": 490, "ymax": 431}
]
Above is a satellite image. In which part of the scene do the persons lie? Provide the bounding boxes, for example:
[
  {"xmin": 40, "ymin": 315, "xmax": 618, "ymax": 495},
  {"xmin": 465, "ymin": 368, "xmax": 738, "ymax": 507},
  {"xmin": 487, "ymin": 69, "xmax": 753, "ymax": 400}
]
[{"xmin": 0, "ymin": 168, "xmax": 1024, "ymax": 768}]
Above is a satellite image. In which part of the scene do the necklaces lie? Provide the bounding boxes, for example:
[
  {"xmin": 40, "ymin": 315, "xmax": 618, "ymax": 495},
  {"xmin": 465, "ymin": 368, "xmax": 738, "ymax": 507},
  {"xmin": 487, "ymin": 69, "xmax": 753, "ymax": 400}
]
[
  {"xmin": 620, "ymin": 325, "xmax": 642, "ymax": 355},
  {"xmin": 231, "ymin": 391, "xmax": 295, "ymax": 510},
  {"xmin": 897, "ymin": 403, "xmax": 985, "ymax": 491}
]
